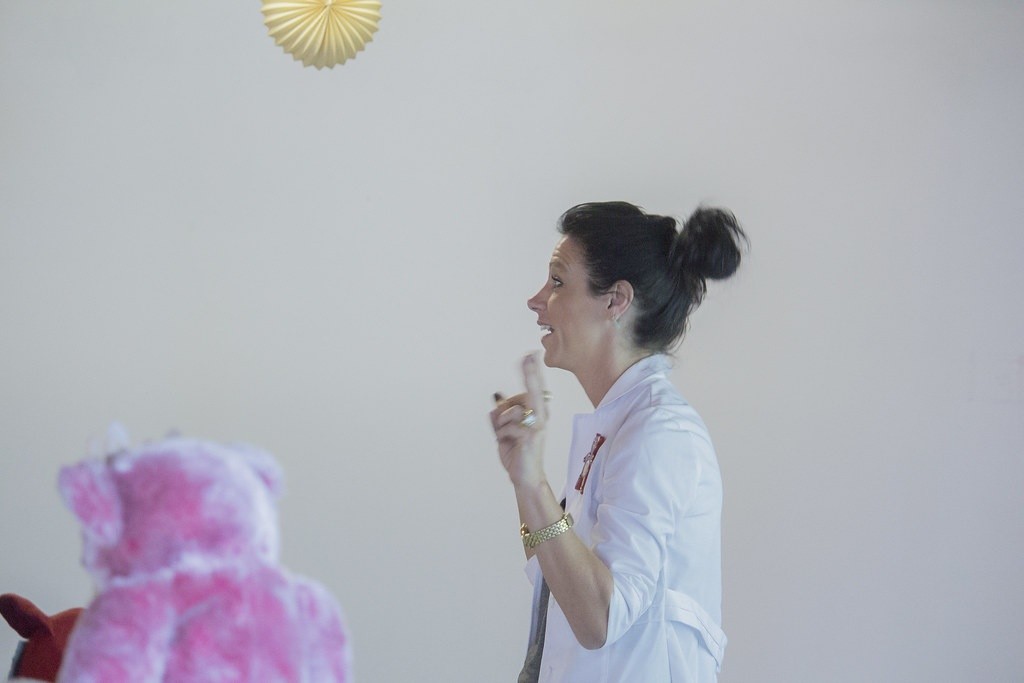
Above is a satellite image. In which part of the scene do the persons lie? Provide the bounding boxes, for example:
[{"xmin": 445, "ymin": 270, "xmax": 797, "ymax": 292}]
[{"xmin": 490, "ymin": 202, "xmax": 752, "ymax": 683}]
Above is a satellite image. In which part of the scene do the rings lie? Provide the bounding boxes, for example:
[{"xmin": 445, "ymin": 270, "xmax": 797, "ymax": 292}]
[
  {"xmin": 521, "ymin": 409, "xmax": 536, "ymax": 428},
  {"xmin": 543, "ymin": 391, "xmax": 550, "ymax": 401}
]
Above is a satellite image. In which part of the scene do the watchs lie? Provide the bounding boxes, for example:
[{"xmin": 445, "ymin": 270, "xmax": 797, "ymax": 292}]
[{"xmin": 521, "ymin": 512, "xmax": 575, "ymax": 551}]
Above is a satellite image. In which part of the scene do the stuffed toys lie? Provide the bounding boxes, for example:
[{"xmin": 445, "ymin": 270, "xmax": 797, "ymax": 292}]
[{"xmin": 0, "ymin": 440, "xmax": 350, "ymax": 683}]
